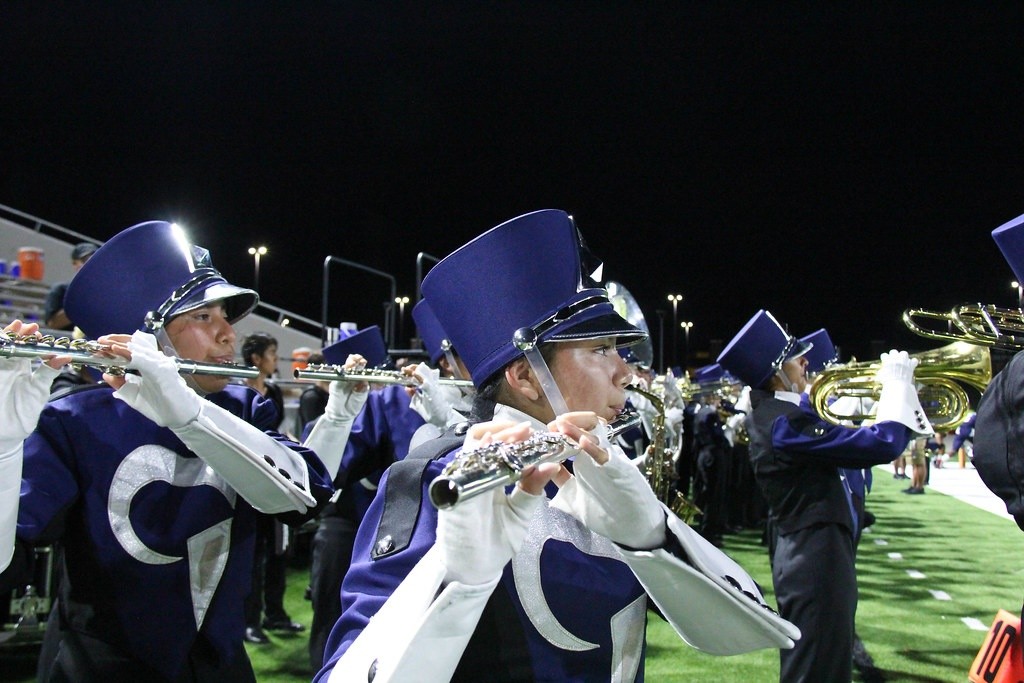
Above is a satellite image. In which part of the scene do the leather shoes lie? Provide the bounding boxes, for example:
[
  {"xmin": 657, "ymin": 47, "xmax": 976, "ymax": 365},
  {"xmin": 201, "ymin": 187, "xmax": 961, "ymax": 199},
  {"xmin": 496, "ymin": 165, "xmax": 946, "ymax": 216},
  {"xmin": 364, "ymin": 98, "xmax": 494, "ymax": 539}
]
[
  {"xmin": 243, "ymin": 626, "xmax": 270, "ymax": 643},
  {"xmin": 263, "ymin": 609, "xmax": 305, "ymax": 630}
]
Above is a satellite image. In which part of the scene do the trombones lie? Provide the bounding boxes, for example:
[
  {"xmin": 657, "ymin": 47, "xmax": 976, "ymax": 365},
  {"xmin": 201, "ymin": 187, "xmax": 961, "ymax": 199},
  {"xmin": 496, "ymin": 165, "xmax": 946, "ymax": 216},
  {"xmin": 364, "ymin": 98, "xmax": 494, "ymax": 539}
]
[{"xmin": 901, "ymin": 302, "xmax": 1024, "ymax": 352}]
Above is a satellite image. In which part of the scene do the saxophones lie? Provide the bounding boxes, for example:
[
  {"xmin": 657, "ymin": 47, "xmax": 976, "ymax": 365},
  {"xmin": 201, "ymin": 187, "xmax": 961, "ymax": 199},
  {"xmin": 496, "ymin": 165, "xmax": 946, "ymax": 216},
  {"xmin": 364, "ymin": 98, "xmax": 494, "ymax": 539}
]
[{"xmin": 623, "ymin": 383, "xmax": 704, "ymax": 528}]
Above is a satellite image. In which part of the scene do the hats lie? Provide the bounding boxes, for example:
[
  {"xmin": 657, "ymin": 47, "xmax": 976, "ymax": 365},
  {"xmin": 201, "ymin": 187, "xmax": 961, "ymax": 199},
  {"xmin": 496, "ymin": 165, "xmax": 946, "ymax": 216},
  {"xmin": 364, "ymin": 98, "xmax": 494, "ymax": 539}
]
[
  {"xmin": 716, "ymin": 310, "xmax": 814, "ymax": 387},
  {"xmin": 72, "ymin": 242, "xmax": 99, "ymax": 258},
  {"xmin": 321, "ymin": 325, "xmax": 398, "ymax": 371},
  {"xmin": 617, "ymin": 346, "xmax": 640, "ymax": 362},
  {"xmin": 420, "ymin": 209, "xmax": 649, "ymax": 390},
  {"xmin": 412, "ymin": 297, "xmax": 454, "ymax": 366},
  {"xmin": 696, "ymin": 366, "xmax": 710, "ymax": 380},
  {"xmin": 63, "ymin": 220, "xmax": 259, "ymax": 340},
  {"xmin": 701, "ymin": 364, "xmax": 725, "ymax": 387},
  {"xmin": 992, "ymin": 214, "xmax": 1024, "ymax": 286},
  {"xmin": 796, "ymin": 328, "xmax": 835, "ymax": 370}
]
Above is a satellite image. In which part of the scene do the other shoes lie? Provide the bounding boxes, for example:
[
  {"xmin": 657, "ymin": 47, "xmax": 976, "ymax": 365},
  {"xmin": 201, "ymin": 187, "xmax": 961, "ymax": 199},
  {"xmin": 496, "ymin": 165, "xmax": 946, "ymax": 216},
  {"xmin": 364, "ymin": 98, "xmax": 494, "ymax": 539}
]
[
  {"xmin": 717, "ymin": 535, "xmax": 723, "ymax": 540},
  {"xmin": 902, "ymin": 487, "xmax": 924, "ymax": 494},
  {"xmin": 900, "ymin": 474, "xmax": 910, "ymax": 478},
  {"xmin": 894, "ymin": 474, "xmax": 901, "ymax": 479},
  {"xmin": 712, "ymin": 540, "xmax": 723, "ymax": 547},
  {"xmin": 722, "ymin": 527, "xmax": 737, "ymax": 535}
]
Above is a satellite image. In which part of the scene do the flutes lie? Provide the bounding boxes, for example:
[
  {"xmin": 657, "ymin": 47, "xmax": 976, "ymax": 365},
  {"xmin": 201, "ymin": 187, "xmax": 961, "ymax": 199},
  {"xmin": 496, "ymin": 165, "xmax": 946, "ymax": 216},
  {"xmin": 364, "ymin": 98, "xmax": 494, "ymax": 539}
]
[
  {"xmin": 293, "ymin": 361, "xmax": 475, "ymax": 388},
  {"xmin": 0, "ymin": 330, "xmax": 261, "ymax": 380},
  {"xmin": 427, "ymin": 412, "xmax": 644, "ymax": 512}
]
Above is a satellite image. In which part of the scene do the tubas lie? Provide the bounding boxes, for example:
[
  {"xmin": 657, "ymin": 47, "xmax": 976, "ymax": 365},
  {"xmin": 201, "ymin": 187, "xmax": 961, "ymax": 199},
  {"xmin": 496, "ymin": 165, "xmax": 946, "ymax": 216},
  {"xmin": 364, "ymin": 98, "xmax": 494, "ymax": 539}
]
[{"xmin": 803, "ymin": 324, "xmax": 994, "ymax": 433}]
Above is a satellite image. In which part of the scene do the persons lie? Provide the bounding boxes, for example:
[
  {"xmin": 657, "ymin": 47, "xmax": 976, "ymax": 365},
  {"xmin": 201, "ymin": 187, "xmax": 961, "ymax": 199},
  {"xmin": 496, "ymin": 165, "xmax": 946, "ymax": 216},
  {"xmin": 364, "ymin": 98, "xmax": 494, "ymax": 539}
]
[
  {"xmin": 0, "ymin": 209, "xmax": 1024, "ymax": 683},
  {"xmin": 716, "ymin": 308, "xmax": 933, "ymax": 683},
  {"xmin": 306, "ymin": 210, "xmax": 803, "ymax": 683},
  {"xmin": 1, "ymin": 210, "xmax": 333, "ymax": 683}
]
